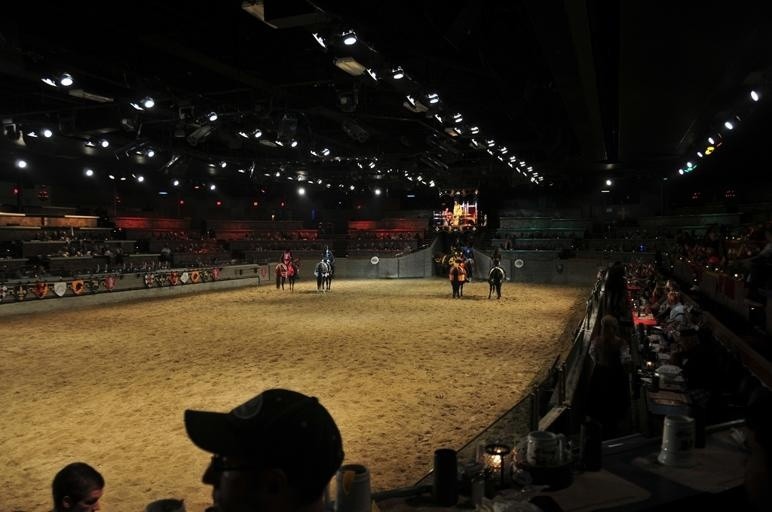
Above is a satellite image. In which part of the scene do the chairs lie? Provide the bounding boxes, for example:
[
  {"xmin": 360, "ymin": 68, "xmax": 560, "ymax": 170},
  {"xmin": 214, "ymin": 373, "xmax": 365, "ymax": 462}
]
[{"xmin": 680, "ymin": 325, "xmax": 772, "ymax": 450}]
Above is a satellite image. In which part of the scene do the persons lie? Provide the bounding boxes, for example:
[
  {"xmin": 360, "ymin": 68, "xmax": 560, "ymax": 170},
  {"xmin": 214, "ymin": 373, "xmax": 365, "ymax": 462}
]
[
  {"xmin": 589, "ymin": 219, "xmax": 772, "ymax": 404},
  {"xmin": 441, "ymin": 207, "xmax": 453, "ymax": 226},
  {"xmin": 185, "ymin": 388, "xmax": 347, "ymax": 511},
  {"xmin": 452, "ymin": 200, "xmax": 463, "ymax": 225},
  {"xmin": 0, "ymin": 225, "xmax": 229, "ymax": 282},
  {"xmin": 487, "ymin": 246, "xmax": 510, "ymax": 282},
  {"xmin": 444, "ymin": 243, "xmax": 474, "ymax": 282},
  {"xmin": 321, "ymin": 244, "xmax": 335, "ymax": 266},
  {"xmin": 282, "ymin": 245, "xmax": 293, "ymax": 267},
  {"xmin": 51, "ymin": 461, "xmax": 106, "ymax": 512},
  {"xmin": 345, "ymin": 228, "xmax": 422, "ymax": 250},
  {"xmin": 492, "ymin": 215, "xmax": 617, "ymax": 255}
]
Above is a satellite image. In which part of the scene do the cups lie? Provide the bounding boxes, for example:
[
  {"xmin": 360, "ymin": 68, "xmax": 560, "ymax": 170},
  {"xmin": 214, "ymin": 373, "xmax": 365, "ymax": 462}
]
[
  {"xmin": 527, "ymin": 431, "xmax": 565, "ymax": 467},
  {"xmin": 658, "ymin": 415, "xmax": 695, "ymax": 468},
  {"xmin": 335, "ymin": 464, "xmax": 372, "ymax": 511}
]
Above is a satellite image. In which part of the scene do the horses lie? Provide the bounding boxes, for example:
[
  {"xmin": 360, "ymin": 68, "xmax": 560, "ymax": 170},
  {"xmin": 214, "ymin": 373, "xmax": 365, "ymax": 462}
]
[
  {"xmin": 449, "ymin": 259, "xmax": 473, "ymax": 298},
  {"xmin": 316, "ymin": 249, "xmax": 337, "ymax": 294},
  {"xmin": 487, "ymin": 266, "xmax": 505, "ymax": 299},
  {"xmin": 275, "ymin": 256, "xmax": 302, "ymax": 292}
]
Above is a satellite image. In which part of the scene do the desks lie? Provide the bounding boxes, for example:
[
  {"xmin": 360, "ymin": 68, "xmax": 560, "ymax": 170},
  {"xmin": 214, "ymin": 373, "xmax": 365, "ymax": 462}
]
[
  {"xmin": 490, "ymin": 213, "xmax": 740, "ymax": 258},
  {"xmin": 341, "ymin": 210, "xmax": 435, "ymax": 258},
  {"xmin": 372, "ymin": 426, "xmax": 771, "ymax": 512},
  {"xmin": 623, "ymin": 271, "xmax": 692, "ymax": 434},
  {"xmin": 1, "ymin": 211, "xmax": 335, "ymax": 277},
  {"xmin": 672, "ymin": 239, "xmax": 770, "ymax": 315}
]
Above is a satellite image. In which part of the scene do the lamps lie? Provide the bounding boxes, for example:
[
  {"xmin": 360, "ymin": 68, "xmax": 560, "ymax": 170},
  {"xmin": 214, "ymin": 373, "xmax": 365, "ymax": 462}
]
[
  {"xmin": 343, "ymin": 30, "xmax": 357, "ymax": 45},
  {"xmin": 59, "ymin": 71, "xmax": 75, "ymax": 86},
  {"xmin": 390, "ymin": 65, "xmax": 404, "ymax": 80}
]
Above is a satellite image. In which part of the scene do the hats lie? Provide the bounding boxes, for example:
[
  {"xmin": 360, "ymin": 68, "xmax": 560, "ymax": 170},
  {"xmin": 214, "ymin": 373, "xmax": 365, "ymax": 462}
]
[{"xmin": 183, "ymin": 388, "xmax": 345, "ymax": 494}]
